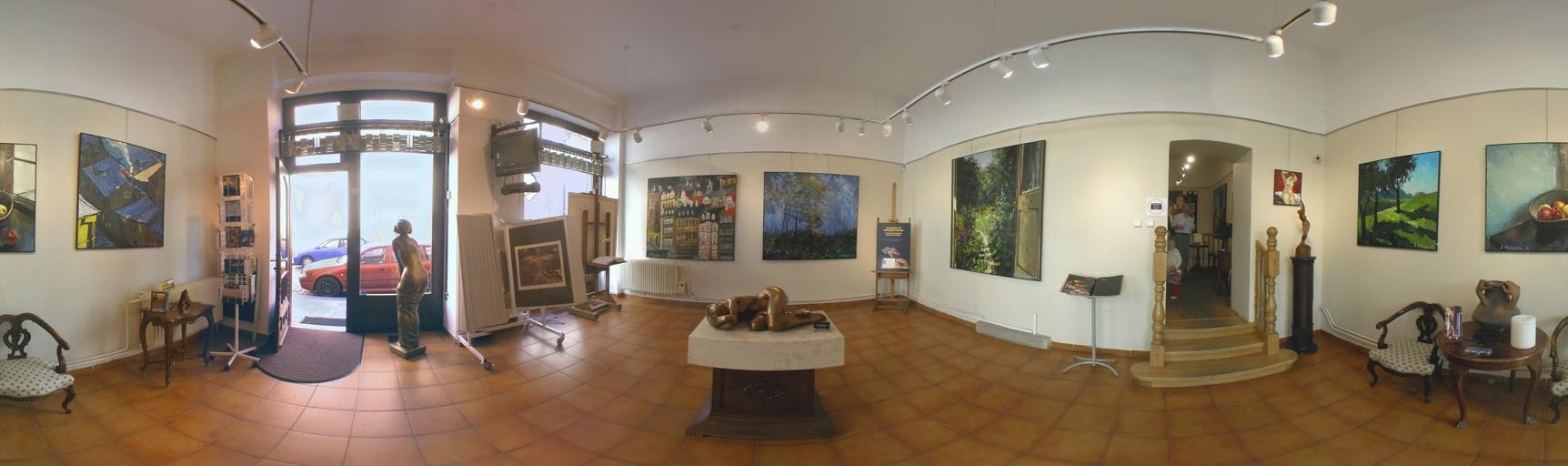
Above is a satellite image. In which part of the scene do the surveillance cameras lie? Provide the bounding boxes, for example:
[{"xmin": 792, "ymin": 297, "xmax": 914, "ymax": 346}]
[
  {"xmin": 1314, "ymin": 153, "xmax": 1322, "ymax": 163},
  {"xmin": 601, "ymin": 154, "xmax": 608, "ymax": 159}
]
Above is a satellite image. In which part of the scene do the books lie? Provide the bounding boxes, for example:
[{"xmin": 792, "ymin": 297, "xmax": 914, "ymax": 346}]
[
  {"xmin": 214, "ymin": 169, "xmax": 260, "ymax": 308},
  {"xmin": 1058, "ymin": 272, "xmax": 1123, "ymax": 298}
]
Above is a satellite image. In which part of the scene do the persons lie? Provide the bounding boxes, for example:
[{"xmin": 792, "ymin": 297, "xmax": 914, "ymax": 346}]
[
  {"xmin": 1475, "ymin": 279, "xmax": 1520, "ymax": 309},
  {"xmin": 1279, "ymin": 171, "xmax": 1298, "ymax": 205},
  {"xmin": 391, "ymin": 217, "xmax": 430, "ymax": 352},
  {"xmin": 1296, "ymin": 200, "xmax": 1310, "ymax": 246},
  {"xmin": 705, "ymin": 284, "xmax": 826, "ymax": 332},
  {"xmin": 1170, "ymin": 202, "xmax": 1194, "ymax": 280},
  {"xmin": 1166, "ymin": 236, "xmax": 1182, "ymax": 301}
]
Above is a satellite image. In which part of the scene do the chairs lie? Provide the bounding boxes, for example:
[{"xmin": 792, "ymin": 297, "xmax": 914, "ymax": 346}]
[
  {"xmin": 1366, "ymin": 301, "xmax": 1446, "ymax": 403},
  {"xmin": 0, "ymin": 312, "xmax": 75, "ymax": 414},
  {"xmin": 1550, "ymin": 316, "xmax": 1568, "ymax": 424}
]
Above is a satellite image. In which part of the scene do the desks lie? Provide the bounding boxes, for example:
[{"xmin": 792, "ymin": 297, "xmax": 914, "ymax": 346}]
[{"xmin": 139, "ymin": 301, "xmax": 215, "ymax": 387}]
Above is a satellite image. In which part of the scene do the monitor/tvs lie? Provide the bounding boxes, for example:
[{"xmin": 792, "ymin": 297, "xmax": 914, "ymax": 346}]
[{"xmin": 492, "ymin": 128, "xmax": 540, "ymax": 177}]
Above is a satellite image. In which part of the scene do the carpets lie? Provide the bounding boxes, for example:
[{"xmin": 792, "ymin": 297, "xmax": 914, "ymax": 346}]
[{"xmin": 252, "ymin": 326, "xmax": 365, "ymax": 384}]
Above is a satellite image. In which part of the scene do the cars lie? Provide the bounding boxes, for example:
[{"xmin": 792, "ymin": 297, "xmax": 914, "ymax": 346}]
[
  {"xmin": 293, "ymin": 237, "xmax": 369, "ymax": 266},
  {"xmin": 298, "ymin": 242, "xmax": 432, "ymax": 297}
]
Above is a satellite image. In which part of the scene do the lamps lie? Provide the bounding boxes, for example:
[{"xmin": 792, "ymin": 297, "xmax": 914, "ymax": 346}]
[
  {"xmin": 597, "ymin": 128, "xmax": 643, "ymax": 143},
  {"xmin": 1264, "ymin": 2, "xmax": 1337, "ymax": 58},
  {"xmin": 835, "ymin": 47, "xmax": 1049, "ymax": 137},
  {"xmin": 700, "ymin": 115, "xmax": 713, "ymax": 133},
  {"xmin": 249, "ymin": 29, "xmax": 306, "ymax": 95},
  {"xmin": 516, "ymin": 97, "xmax": 528, "ymax": 116}
]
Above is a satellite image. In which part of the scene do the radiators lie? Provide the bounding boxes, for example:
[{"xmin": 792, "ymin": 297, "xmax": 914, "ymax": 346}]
[{"xmin": 621, "ymin": 258, "xmax": 686, "ymax": 297}]
